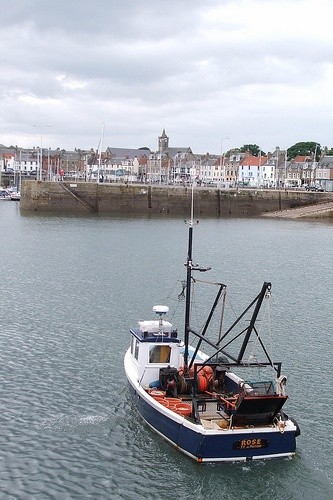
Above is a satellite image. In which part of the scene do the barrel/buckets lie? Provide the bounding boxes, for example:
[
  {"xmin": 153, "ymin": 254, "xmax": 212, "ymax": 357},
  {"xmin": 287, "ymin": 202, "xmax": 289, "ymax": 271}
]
[{"xmin": 147, "ymin": 389, "xmax": 192, "ymax": 419}]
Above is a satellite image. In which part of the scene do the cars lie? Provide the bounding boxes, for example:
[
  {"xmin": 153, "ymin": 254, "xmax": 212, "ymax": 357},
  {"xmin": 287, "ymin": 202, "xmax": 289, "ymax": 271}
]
[{"xmin": 305, "ymin": 183, "xmax": 324, "ymax": 192}]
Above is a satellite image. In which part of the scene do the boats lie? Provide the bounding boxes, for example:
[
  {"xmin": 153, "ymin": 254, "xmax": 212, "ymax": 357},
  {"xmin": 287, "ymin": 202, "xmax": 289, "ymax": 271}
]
[{"xmin": 124, "ymin": 185, "xmax": 302, "ymax": 464}]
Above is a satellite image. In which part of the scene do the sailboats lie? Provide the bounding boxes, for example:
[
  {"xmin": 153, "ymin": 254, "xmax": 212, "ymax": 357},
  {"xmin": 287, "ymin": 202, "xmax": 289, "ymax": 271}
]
[{"xmin": 0, "ymin": 143, "xmax": 62, "ymax": 202}]
